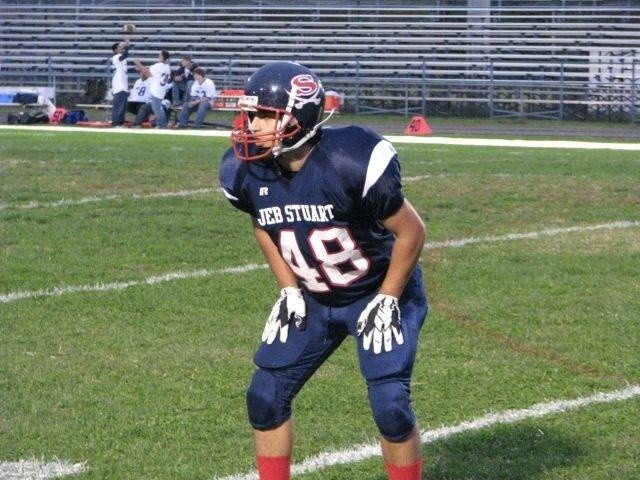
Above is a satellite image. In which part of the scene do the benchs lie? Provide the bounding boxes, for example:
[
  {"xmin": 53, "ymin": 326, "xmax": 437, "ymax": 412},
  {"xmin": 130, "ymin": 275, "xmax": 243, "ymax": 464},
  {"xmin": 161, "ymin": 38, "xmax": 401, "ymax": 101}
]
[{"xmin": 1, "ymin": 1, "xmax": 639, "ymax": 131}]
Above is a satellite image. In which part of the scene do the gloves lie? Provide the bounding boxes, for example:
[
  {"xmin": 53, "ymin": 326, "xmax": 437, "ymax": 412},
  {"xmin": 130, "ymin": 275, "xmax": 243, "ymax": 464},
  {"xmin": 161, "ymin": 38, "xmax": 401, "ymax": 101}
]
[
  {"xmin": 262, "ymin": 287, "xmax": 307, "ymax": 344},
  {"xmin": 355, "ymin": 293, "xmax": 404, "ymax": 355}
]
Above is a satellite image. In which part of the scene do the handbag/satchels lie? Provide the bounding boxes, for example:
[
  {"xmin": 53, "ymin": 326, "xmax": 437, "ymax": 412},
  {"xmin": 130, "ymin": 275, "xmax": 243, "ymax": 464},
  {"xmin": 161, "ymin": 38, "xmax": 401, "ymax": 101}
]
[
  {"xmin": 12, "ymin": 93, "xmax": 38, "ymax": 103},
  {"xmin": 8, "ymin": 108, "xmax": 84, "ymax": 125}
]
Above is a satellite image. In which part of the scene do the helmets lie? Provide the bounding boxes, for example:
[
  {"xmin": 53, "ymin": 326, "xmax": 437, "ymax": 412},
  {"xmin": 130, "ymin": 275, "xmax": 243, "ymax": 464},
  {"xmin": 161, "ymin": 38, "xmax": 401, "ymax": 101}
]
[{"xmin": 231, "ymin": 62, "xmax": 326, "ymax": 163}]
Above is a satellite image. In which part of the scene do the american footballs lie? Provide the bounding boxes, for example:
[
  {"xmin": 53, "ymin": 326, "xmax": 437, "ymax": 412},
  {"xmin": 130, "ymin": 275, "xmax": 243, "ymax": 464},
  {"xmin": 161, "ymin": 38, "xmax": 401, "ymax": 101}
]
[{"xmin": 123, "ymin": 23, "xmax": 136, "ymax": 32}]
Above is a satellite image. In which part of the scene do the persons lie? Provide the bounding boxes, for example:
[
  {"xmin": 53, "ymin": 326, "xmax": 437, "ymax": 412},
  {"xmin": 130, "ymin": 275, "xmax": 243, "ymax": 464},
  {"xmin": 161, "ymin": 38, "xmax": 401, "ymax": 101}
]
[
  {"xmin": 103, "ymin": 36, "xmax": 215, "ymax": 130},
  {"xmin": 217, "ymin": 59, "xmax": 431, "ymax": 480}
]
